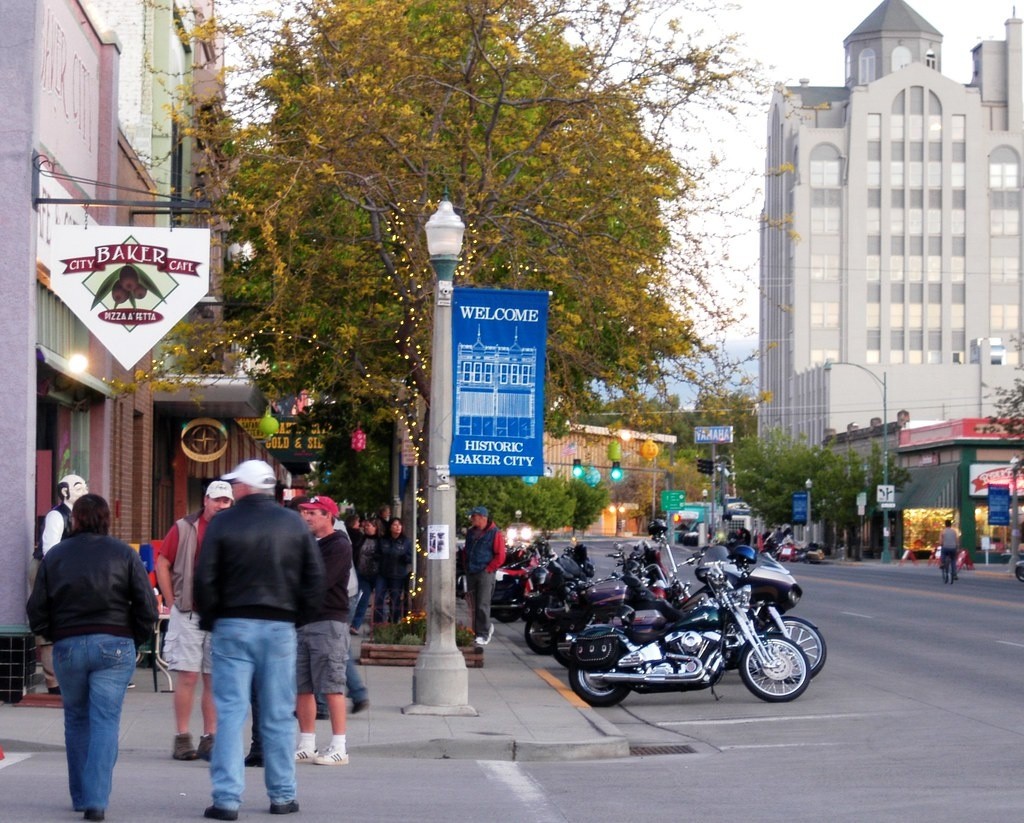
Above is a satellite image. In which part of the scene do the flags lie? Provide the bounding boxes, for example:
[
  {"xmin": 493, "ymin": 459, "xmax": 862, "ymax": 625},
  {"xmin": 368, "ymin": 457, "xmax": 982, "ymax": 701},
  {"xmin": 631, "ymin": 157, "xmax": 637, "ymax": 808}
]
[{"xmin": 560, "ymin": 441, "xmax": 577, "ymax": 456}]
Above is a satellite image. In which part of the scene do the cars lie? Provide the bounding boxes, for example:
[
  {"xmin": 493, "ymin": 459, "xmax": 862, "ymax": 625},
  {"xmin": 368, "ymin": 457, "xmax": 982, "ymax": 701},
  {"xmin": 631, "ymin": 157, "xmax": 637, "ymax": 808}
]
[{"xmin": 508, "ymin": 523, "xmax": 532, "ymax": 545}]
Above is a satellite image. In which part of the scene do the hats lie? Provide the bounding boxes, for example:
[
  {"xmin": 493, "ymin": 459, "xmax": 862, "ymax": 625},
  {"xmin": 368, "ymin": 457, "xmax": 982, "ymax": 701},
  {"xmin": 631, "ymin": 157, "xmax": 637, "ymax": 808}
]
[
  {"xmin": 205, "ymin": 481, "xmax": 233, "ymax": 500},
  {"xmin": 297, "ymin": 496, "xmax": 340, "ymax": 520},
  {"xmin": 467, "ymin": 506, "xmax": 490, "ymax": 518},
  {"xmin": 220, "ymin": 459, "xmax": 277, "ymax": 490}
]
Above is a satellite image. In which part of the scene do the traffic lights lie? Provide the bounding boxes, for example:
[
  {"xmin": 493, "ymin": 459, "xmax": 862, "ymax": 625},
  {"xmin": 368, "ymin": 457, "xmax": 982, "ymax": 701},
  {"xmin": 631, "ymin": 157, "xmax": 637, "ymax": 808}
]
[
  {"xmin": 673, "ymin": 512, "xmax": 680, "ymax": 522},
  {"xmin": 573, "ymin": 458, "xmax": 581, "ymax": 479},
  {"xmin": 612, "ymin": 461, "xmax": 620, "ymax": 480}
]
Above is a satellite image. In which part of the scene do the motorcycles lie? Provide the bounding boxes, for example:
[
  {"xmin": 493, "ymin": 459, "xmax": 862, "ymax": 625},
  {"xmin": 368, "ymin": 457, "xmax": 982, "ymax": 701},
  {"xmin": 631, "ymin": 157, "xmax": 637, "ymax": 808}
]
[
  {"xmin": 569, "ymin": 544, "xmax": 811, "ymax": 709},
  {"xmin": 493, "ymin": 519, "xmax": 827, "ymax": 682}
]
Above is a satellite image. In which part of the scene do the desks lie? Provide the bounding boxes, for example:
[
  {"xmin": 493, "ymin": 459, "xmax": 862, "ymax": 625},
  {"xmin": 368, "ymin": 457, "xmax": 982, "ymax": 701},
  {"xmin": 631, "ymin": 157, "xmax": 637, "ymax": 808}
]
[{"xmin": 135, "ymin": 614, "xmax": 172, "ymax": 693}]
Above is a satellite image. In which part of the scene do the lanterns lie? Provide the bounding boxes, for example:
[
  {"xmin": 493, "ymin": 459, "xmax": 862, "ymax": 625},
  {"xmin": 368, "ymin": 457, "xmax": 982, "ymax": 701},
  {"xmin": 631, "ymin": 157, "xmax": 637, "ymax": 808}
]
[
  {"xmin": 585, "ymin": 467, "xmax": 603, "ymax": 487},
  {"xmin": 611, "ymin": 468, "xmax": 623, "ymax": 481},
  {"xmin": 572, "ymin": 465, "xmax": 585, "ymax": 478},
  {"xmin": 640, "ymin": 435, "xmax": 662, "ymax": 461},
  {"xmin": 607, "ymin": 434, "xmax": 623, "ymax": 462},
  {"xmin": 523, "ymin": 467, "xmax": 539, "ymax": 485}
]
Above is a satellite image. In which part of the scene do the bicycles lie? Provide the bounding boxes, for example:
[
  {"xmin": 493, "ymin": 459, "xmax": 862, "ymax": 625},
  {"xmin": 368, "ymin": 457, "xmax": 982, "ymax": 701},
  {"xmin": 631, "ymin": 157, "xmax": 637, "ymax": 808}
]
[{"xmin": 941, "ymin": 552, "xmax": 955, "ymax": 584}]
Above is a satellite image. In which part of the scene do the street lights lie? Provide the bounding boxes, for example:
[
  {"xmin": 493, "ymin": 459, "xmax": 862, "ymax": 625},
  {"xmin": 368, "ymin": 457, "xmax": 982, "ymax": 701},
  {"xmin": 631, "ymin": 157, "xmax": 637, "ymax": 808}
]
[
  {"xmin": 703, "ymin": 489, "xmax": 708, "ymax": 548},
  {"xmin": 804, "ymin": 478, "xmax": 812, "ymax": 547},
  {"xmin": 825, "ymin": 358, "xmax": 892, "ymax": 564},
  {"xmin": 412, "ymin": 183, "xmax": 471, "ymax": 708},
  {"xmin": 1008, "ymin": 455, "xmax": 1021, "ymax": 574}
]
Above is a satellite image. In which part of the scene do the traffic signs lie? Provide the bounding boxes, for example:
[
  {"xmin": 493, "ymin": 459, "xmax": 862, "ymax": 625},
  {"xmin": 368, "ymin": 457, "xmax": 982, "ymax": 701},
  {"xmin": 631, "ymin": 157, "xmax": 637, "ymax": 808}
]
[{"xmin": 661, "ymin": 490, "xmax": 686, "ymax": 511}]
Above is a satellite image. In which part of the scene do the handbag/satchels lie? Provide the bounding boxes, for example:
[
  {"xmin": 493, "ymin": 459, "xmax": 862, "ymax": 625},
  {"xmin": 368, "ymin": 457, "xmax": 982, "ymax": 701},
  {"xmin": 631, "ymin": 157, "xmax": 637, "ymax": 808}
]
[{"xmin": 935, "ymin": 546, "xmax": 942, "ymax": 559}]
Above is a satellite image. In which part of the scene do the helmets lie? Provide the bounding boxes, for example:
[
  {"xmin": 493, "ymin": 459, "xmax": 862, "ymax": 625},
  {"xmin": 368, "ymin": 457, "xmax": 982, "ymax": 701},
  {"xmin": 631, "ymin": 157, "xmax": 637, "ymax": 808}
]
[
  {"xmin": 531, "ymin": 566, "xmax": 551, "ymax": 585},
  {"xmin": 648, "ymin": 519, "xmax": 669, "ymax": 538},
  {"xmin": 728, "ymin": 544, "xmax": 758, "ymax": 565}
]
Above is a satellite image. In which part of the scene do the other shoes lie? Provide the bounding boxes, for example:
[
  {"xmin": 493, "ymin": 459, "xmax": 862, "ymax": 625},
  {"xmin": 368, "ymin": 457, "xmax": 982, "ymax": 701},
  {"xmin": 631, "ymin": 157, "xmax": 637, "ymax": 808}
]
[
  {"xmin": 350, "ymin": 700, "xmax": 369, "ymax": 715},
  {"xmin": 474, "ymin": 623, "xmax": 494, "ymax": 645},
  {"xmin": 954, "ymin": 575, "xmax": 958, "ymax": 580},
  {"xmin": 244, "ymin": 751, "xmax": 264, "ymax": 767},
  {"xmin": 203, "ymin": 805, "xmax": 238, "ymax": 821},
  {"xmin": 83, "ymin": 808, "xmax": 106, "ymax": 819},
  {"xmin": 269, "ymin": 799, "xmax": 301, "ymax": 816},
  {"xmin": 292, "ymin": 709, "xmax": 329, "ymax": 721},
  {"xmin": 939, "ymin": 564, "xmax": 945, "ymax": 569},
  {"xmin": 349, "ymin": 627, "xmax": 360, "ymax": 637}
]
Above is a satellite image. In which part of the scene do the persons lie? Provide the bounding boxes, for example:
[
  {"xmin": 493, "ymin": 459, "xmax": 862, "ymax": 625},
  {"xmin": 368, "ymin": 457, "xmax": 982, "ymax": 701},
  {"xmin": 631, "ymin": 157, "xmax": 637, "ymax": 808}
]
[
  {"xmin": 23, "ymin": 493, "xmax": 156, "ymax": 823},
  {"xmin": 938, "ymin": 519, "xmax": 958, "ymax": 585},
  {"xmin": 152, "ymin": 481, "xmax": 232, "ymax": 763},
  {"xmin": 35, "ymin": 472, "xmax": 115, "ymax": 692},
  {"xmin": 465, "ymin": 506, "xmax": 506, "ymax": 646},
  {"xmin": 290, "ymin": 496, "xmax": 414, "ymax": 764},
  {"xmin": 200, "ymin": 460, "xmax": 299, "ymax": 823}
]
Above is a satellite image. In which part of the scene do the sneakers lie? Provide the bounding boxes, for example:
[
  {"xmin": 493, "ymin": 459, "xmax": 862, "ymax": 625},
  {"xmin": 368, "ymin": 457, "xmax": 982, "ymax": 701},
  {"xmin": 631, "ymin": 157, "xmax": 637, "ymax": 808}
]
[
  {"xmin": 172, "ymin": 732, "xmax": 198, "ymax": 762},
  {"xmin": 292, "ymin": 744, "xmax": 319, "ymax": 764},
  {"xmin": 198, "ymin": 733, "xmax": 216, "ymax": 762},
  {"xmin": 310, "ymin": 747, "xmax": 350, "ymax": 766}
]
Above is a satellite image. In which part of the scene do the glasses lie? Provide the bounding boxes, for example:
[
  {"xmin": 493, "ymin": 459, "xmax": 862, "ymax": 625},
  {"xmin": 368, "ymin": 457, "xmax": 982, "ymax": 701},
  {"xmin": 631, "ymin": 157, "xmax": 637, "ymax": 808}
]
[{"xmin": 309, "ymin": 498, "xmax": 330, "ymax": 508}]
